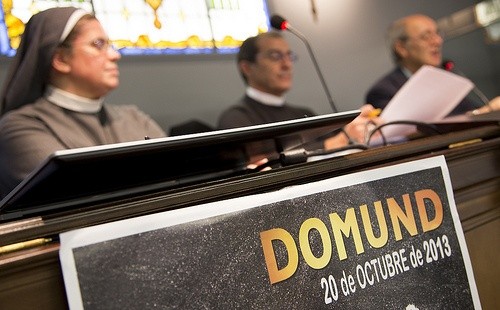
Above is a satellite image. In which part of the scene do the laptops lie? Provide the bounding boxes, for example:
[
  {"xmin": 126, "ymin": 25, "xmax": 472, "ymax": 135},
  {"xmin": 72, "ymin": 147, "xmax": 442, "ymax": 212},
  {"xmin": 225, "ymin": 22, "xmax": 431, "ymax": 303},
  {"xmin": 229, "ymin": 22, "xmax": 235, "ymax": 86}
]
[{"xmin": 6, "ymin": 111, "xmax": 361, "ymax": 225}]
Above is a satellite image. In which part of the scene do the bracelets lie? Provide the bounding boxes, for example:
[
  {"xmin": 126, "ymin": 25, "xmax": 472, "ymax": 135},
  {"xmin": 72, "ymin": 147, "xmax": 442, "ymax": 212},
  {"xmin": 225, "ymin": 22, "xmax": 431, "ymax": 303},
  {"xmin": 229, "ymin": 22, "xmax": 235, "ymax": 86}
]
[{"xmin": 472, "ymin": 110, "xmax": 480, "ymax": 116}]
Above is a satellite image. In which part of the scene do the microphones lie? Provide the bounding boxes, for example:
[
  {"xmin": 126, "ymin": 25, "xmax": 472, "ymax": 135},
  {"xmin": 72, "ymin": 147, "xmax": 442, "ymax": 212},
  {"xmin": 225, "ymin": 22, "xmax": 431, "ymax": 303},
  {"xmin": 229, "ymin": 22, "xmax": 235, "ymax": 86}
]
[
  {"xmin": 272, "ymin": 14, "xmax": 365, "ymax": 157},
  {"xmin": 444, "ymin": 60, "xmax": 495, "ymax": 114}
]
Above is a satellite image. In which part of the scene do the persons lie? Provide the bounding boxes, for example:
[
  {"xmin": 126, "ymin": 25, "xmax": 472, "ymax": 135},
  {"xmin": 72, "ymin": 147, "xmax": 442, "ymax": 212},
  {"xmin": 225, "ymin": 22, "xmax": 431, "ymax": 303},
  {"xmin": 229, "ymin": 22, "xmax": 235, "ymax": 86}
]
[
  {"xmin": 216, "ymin": 32, "xmax": 382, "ymax": 152},
  {"xmin": 0, "ymin": 1, "xmax": 169, "ymax": 201},
  {"xmin": 365, "ymin": 14, "xmax": 500, "ymax": 140}
]
